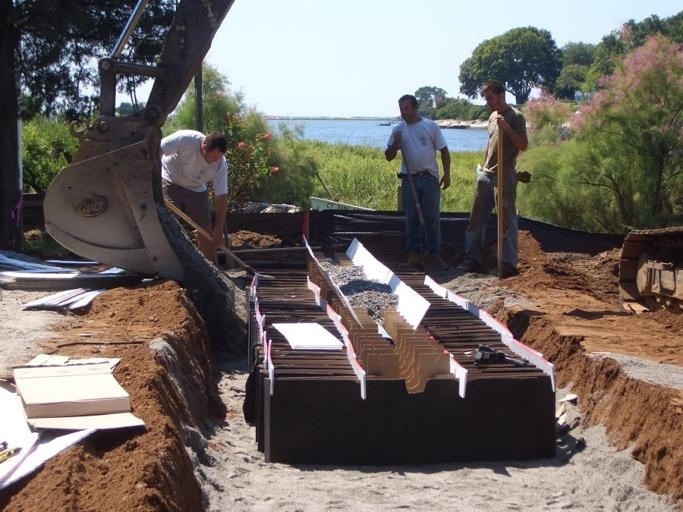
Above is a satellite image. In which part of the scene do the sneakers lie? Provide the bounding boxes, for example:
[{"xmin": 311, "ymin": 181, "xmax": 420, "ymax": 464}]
[
  {"xmin": 408, "ymin": 251, "xmax": 449, "ymax": 270},
  {"xmin": 456, "ymin": 263, "xmax": 472, "ymax": 271},
  {"xmin": 489, "ymin": 263, "xmax": 520, "ymax": 276}
]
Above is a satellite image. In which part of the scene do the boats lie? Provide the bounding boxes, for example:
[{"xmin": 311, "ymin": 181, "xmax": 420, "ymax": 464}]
[
  {"xmin": 308, "ymin": 195, "xmax": 376, "ymax": 211},
  {"xmin": 440, "ymin": 124, "xmax": 469, "ymax": 129},
  {"xmin": 379, "ymin": 121, "xmax": 391, "ymax": 126}
]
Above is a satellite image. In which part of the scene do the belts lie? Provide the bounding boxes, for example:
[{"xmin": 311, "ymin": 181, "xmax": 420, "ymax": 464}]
[{"xmin": 397, "ymin": 173, "xmax": 416, "ymax": 178}]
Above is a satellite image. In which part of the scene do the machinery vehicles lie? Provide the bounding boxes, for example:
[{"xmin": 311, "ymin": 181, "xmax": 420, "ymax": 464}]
[{"xmin": 0, "ymin": 0, "xmax": 248, "ymax": 353}]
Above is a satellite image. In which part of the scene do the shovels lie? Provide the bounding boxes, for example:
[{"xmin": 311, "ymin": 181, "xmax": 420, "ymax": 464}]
[
  {"xmin": 165, "ymin": 201, "xmax": 276, "ymax": 281},
  {"xmin": 399, "ymin": 143, "xmax": 440, "ymax": 274}
]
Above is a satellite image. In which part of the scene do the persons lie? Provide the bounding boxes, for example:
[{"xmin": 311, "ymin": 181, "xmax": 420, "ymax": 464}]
[
  {"xmin": 385, "ymin": 94, "xmax": 451, "ymax": 270},
  {"xmin": 456, "ymin": 80, "xmax": 528, "ymax": 278},
  {"xmin": 161, "ymin": 130, "xmax": 229, "ymax": 264}
]
